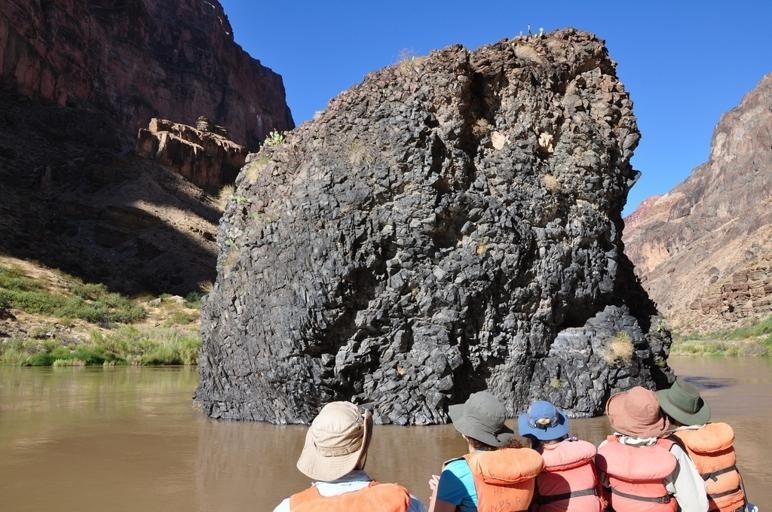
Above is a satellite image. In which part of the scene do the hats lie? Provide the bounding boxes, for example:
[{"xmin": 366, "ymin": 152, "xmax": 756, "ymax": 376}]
[
  {"xmin": 294, "ymin": 399, "xmax": 373, "ymax": 482},
  {"xmin": 447, "ymin": 389, "xmax": 516, "ymax": 448},
  {"xmin": 517, "ymin": 398, "xmax": 570, "ymax": 441},
  {"xmin": 605, "ymin": 384, "xmax": 671, "ymax": 440},
  {"xmin": 655, "ymin": 378, "xmax": 712, "ymax": 426}
]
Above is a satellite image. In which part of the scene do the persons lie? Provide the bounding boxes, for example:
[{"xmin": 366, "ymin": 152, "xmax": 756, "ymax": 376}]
[
  {"xmin": 594, "ymin": 385, "xmax": 710, "ymax": 511},
  {"xmin": 426, "ymin": 391, "xmax": 544, "ymax": 512},
  {"xmin": 517, "ymin": 400, "xmax": 608, "ymax": 511},
  {"xmin": 274, "ymin": 399, "xmax": 425, "ymax": 512},
  {"xmin": 654, "ymin": 377, "xmax": 757, "ymax": 512}
]
